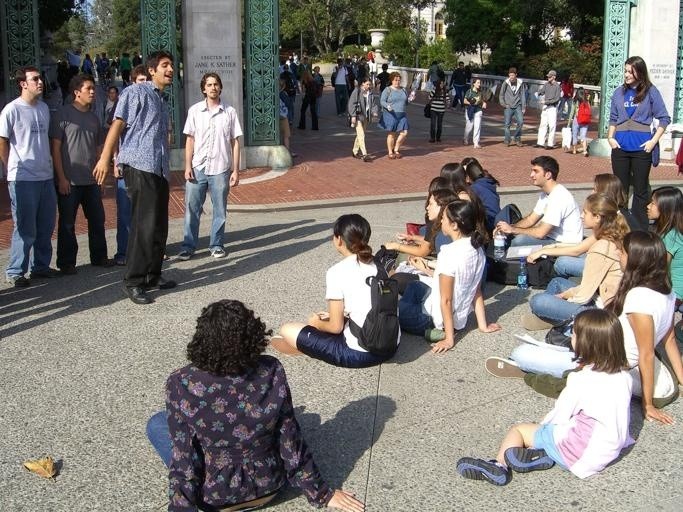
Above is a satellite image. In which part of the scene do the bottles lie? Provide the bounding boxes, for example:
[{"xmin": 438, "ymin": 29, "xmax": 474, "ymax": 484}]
[
  {"xmin": 493, "ymin": 230, "xmax": 505, "ymax": 262},
  {"xmin": 408, "ymin": 86, "xmax": 417, "ymax": 102},
  {"xmin": 516, "ymin": 257, "xmax": 528, "ymax": 290}
]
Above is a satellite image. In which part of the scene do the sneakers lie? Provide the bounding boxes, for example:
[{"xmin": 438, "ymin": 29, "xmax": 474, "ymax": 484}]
[
  {"xmin": 270, "ymin": 336, "xmax": 302, "ymax": 355},
  {"xmin": 521, "ymin": 315, "xmax": 558, "ymax": 330},
  {"xmin": 179, "ymin": 251, "xmax": 191, "ymax": 260},
  {"xmin": 456, "ymin": 457, "xmax": 508, "ymax": 486},
  {"xmin": 485, "ymin": 357, "xmax": 527, "ymax": 378},
  {"xmin": 6, "ymin": 275, "xmax": 28, "ymax": 287},
  {"xmin": 504, "ymin": 447, "xmax": 554, "ymax": 473},
  {"xmin": 30, "ymin": 269, "xmax": 57, "ymax": 277},
  {"xmin": 209, "ymin": 247, "xmax": 225, "ymax": 257}
]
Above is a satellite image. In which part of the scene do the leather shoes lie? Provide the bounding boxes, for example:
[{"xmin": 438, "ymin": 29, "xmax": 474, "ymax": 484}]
[
  {"xmin": 61, "ymin": 265, "xmax": 75, "ymax": 274},
  {"xmin": 122, "ymin": 285, "xmax": 150, "ymax": 303},
  {"xmin": 93, "ymin": 259, "xmax": 126, "ymax": 267},
  {"xmin": 147, "ymin": 278, "xmax": 177, "ymax": 290}
]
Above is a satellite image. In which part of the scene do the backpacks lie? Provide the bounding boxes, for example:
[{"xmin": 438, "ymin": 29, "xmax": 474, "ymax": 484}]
[{"xmin": 576, "ymin": 99, "xmax": 591, "ymax": 124}]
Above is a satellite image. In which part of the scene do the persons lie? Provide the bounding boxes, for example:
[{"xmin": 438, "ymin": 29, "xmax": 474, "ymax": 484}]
[
  {"xmin": 1, "ymin": 46, "xmax": 179, "ymax": 306},
  {"xmin": 427, "ymin": 61, "xmax": 591, "ymax": 157},
  {"xmin": 178, "ymin": 72, "xmax": 243, "ymax": 261},
  {"xmin": 275, "ymin": 50, "xmax": 416, "ymax": 162},
  {"xmin": 608, "ymin": 57, "xmax": 671, "ymax": 229},
  {"xmin": 459, "ymin": 157, "xmax": 683, "ymax": 486},
  {"xmin": 146, "ymin": 298, "xmax": 366, "ymax": 511},
  {"xmin": 273, "ymin": 156, "xmax": 514, "ymax": 367}
]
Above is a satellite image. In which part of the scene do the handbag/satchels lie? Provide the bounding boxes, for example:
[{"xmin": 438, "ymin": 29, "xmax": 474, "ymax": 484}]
[
  {"xmin": 424, "ymin": 104, "xmax": 430, "ymax": 118},
  {"xmin": 562, "ymin": 128, "xmax": 571, "ymax": 149},
  {"xmin": 346, "ymin": 103, "xmax": 361, "ymax": 127},
  {"xmin": 651, "ymin": 128, "xmax": 659, "ymax": 167},
  {"xmin": 496, "ymin": 259, "xmax": 555, "ymax": 290},
  {"xmin": 545, "ymin": 319, "xmax": 573, "ymax": 347},
  {"xmin": 358, "ymin": 275, "xmax": 399, "ymax": 356}
]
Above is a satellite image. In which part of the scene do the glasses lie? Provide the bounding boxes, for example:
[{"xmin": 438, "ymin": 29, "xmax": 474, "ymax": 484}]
[{"xmin": 27, "ymin": 75, "xmax": 41, "ymax": 81}]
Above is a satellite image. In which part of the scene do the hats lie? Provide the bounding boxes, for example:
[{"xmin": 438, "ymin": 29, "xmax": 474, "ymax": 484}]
[{"xmin": 546, "ymin": 70, "xmax": 556, "ymax": 77}]
